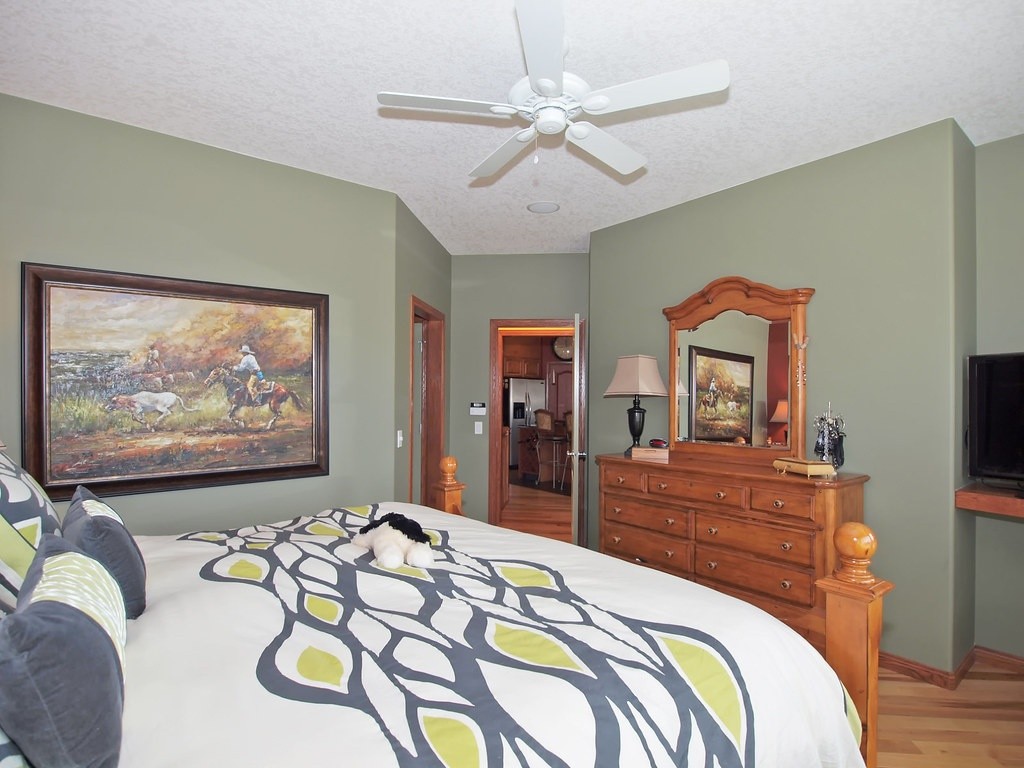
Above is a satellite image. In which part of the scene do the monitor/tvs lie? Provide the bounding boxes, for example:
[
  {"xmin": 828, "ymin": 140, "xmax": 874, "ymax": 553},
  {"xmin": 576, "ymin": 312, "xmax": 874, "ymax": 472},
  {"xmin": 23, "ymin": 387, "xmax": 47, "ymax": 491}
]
[{"xmin": 967, "ymin": 352, "xmax": 1024, "ymax": 500}]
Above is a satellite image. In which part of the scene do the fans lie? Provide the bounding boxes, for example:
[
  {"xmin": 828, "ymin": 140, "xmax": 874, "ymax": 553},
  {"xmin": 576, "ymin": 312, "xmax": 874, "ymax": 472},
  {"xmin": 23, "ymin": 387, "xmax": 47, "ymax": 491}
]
[{"xmin": 377, "ymin": 0, "xmax": 731, "ymax": 178}]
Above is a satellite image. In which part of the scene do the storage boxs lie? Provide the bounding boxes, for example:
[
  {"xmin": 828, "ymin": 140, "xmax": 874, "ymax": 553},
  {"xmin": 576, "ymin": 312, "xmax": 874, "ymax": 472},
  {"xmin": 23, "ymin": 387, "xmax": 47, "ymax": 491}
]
[
  {"xmin": 771, "ymin": 456, "xmax": 835, "ymax": 478},
  {"xmin": 631, "ymin": 446, "xmax": 670, "ymax": 461}
]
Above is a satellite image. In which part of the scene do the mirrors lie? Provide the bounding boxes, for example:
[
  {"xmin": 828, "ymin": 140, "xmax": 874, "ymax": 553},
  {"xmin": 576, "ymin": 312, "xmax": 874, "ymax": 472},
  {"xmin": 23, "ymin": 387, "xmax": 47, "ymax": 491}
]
[{"xmin": 661, "ymin": 276, "xmax": 817, "ymax": 467}]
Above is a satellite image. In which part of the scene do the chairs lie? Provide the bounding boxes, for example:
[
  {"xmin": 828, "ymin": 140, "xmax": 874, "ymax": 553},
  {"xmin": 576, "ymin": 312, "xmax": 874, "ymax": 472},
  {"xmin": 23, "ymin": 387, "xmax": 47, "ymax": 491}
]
[
  {"xmin": 560, "ymin": 412, "xmax": 574, "ymax": 493},
  {"xmin": 533, "ymin": 409, "xmax": 567, "ymax": 489}
]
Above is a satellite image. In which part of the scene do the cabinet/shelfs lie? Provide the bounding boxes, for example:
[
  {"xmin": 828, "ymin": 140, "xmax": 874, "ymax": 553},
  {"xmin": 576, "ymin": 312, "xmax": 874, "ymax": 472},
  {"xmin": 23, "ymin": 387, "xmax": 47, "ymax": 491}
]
[
  {"xmin": 517, "ymin": 422, "xmax": 567, "ymax": 486},
  {"xmin": 595, "ymin": 446, "xmax": 871, "ymax": 661}
]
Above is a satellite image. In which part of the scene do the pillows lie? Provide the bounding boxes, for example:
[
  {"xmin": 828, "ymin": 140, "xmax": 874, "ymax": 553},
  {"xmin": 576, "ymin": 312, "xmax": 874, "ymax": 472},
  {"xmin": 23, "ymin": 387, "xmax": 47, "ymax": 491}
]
[
  {"xmin": 0, "ymin": 450, "xmax": 64, "ymax": 618},
  {"xmin": 1, "ymin": 531, "xmax": 128, "ymax": 768},
  {"xmin": 0, "ymin": 727, "xmax": 32, "ymax": 768},
  {"xmin": 60, "ymin": 485, "xmax": 147, "ymax": 620}
]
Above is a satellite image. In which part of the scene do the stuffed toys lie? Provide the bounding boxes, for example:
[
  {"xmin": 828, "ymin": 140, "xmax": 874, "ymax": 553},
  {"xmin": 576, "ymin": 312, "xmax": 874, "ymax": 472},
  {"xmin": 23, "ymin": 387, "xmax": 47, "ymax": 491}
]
[{"xmin": 353, "ymin": 513, "xmax": 434, "ymax": 569}]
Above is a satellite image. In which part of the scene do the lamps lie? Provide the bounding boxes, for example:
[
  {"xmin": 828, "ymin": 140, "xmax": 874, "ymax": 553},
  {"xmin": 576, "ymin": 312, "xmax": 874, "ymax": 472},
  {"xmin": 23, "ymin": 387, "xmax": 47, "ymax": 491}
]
[{"xmin": 602, "ymin": 354, "xmax": 669, "ymax": 456}]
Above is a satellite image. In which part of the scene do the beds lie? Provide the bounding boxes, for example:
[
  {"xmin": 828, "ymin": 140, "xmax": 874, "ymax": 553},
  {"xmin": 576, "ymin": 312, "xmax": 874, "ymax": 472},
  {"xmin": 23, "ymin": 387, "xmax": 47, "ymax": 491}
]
[{"xmin": 118, "ymin": 501, "xmax": 897, "ymax": 768}]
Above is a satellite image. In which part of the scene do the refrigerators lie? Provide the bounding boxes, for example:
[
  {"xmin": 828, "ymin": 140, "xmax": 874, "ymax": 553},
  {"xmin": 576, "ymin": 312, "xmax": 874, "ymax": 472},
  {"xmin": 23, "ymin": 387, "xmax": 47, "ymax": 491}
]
[{"xmin": 502, "ymin": 376, "xmax": 546, "ymax": 470}]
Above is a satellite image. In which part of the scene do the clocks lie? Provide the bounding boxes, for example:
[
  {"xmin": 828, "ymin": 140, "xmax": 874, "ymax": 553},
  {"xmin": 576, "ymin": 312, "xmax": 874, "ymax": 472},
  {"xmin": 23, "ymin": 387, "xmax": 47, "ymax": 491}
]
[{"xmin": 551, "ymin": 336, "xmax": 573, "ymax": 361}]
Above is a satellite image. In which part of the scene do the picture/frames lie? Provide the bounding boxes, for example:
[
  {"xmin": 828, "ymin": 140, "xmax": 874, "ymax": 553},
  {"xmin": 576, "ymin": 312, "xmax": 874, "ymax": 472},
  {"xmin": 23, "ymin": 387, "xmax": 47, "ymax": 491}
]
[
  {"xmin": 21, "ymin": 259, "xmax": 331, "ymax": 502},
  {"xmin": 688, "ymin": 344, "xmax": 755, "ymax": 447}
]
